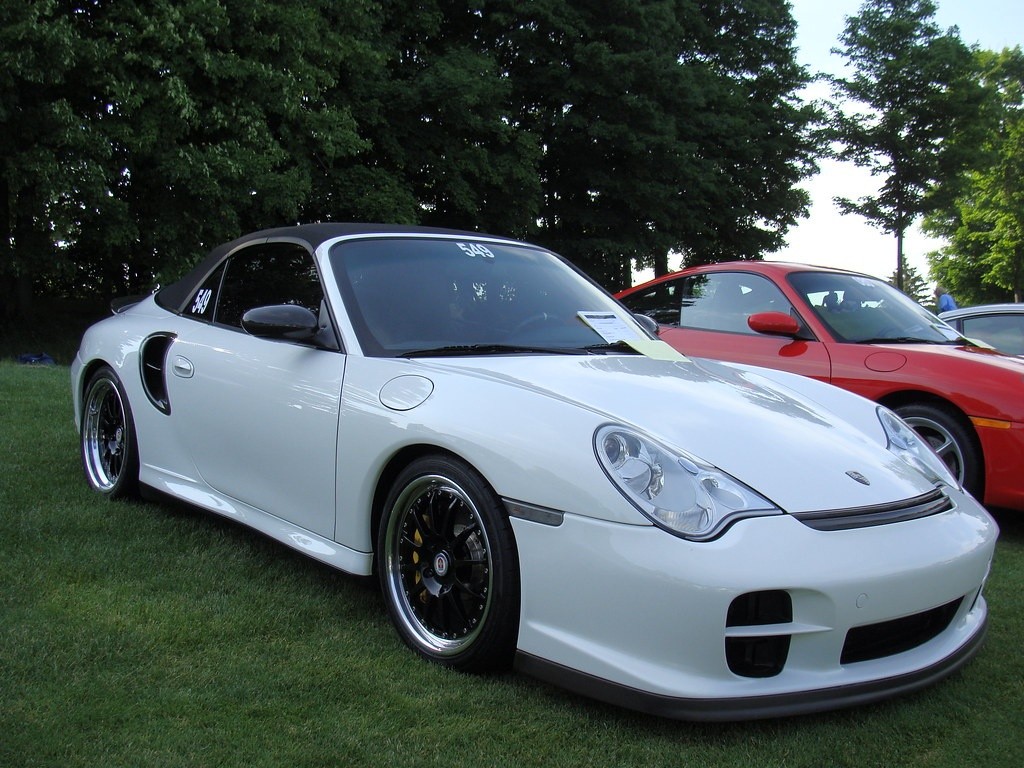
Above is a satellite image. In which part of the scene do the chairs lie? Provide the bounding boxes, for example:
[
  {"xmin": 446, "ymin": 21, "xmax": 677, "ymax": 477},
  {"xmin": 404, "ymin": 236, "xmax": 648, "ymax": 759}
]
[{"xmin": 709, "ymin": 282, "xmax": 746, "ymax": 333}]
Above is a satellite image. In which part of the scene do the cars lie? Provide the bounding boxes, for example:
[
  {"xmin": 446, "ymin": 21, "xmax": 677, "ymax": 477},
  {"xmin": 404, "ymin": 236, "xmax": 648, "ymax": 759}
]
[
  {"xmin": 71, "ymin": 222, "xmax": 1000, "ymax": 723},
  {"xmin": 613, "ymin": 261, "xmax": 1024, "ymax": 511},
  {"xmin": 905, "ymin": 303, "xmax": 1024, "ymax": 354}
]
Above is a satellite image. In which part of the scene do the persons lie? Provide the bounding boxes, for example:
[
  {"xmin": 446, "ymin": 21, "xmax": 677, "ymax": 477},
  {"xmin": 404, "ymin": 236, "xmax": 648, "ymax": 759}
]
[
  {"xmin": 821, "ymin": 290, "xmax": 862, "ymax": 309},
  {"xmin": 935, "ymin": 288, "xmax": 957, "ymax": 311}
]
